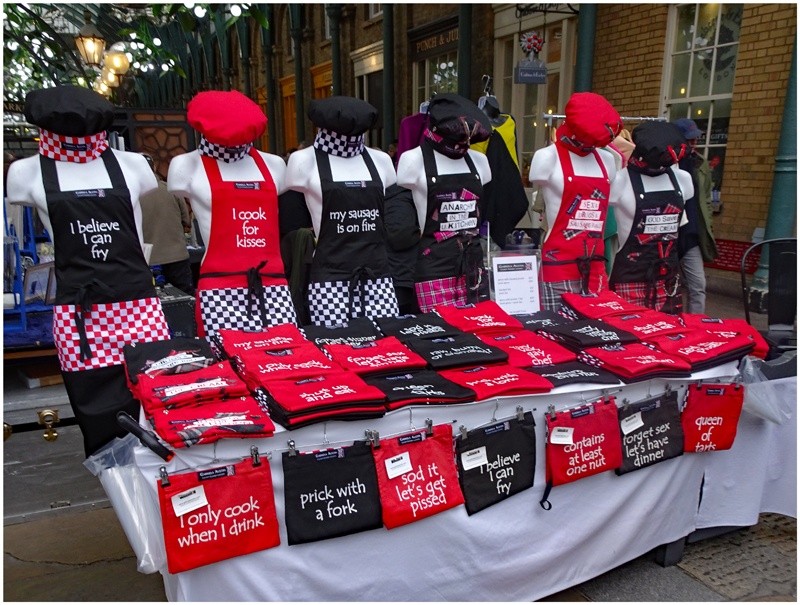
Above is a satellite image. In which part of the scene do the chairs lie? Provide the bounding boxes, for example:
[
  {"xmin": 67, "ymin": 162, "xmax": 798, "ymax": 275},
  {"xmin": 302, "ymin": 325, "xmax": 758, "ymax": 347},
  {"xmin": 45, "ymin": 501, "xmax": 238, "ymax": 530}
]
[{"xmin": 740, "ymin": 237, "xmax": 796, "ymax": 349}]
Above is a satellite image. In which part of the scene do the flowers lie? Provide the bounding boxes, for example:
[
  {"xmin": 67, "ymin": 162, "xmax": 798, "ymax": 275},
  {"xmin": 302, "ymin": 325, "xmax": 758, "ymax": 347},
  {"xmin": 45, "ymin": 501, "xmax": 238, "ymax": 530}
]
[{"xmin": 519, "ymin": 30, "xmax": 545, "ymax": 56}]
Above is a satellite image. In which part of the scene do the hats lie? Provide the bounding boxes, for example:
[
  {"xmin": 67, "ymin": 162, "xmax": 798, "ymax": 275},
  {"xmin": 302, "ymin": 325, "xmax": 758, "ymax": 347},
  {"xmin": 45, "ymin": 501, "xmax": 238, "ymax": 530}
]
[{"xmin": 673, "ymin": 118, "xmax": 706, "ymax": 140}]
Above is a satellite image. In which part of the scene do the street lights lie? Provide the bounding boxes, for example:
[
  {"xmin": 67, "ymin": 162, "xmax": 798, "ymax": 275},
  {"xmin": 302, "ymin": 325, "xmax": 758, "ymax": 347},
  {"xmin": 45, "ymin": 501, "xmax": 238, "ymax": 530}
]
[{"xmin": 74, "ymin": 10, "xmax": 136, "ymax": 105}]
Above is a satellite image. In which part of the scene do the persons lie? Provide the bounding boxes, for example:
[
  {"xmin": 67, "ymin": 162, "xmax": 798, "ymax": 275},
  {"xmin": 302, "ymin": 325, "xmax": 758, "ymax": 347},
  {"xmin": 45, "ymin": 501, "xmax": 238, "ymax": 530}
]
[
  {"xmin": 672, "ymin": 118, "xmax": 721, "ymax": 317},
  {"xmin": 134, "ymin": 152, "xmax": 198, "ymax": 298},
  {"xmin": 166, "ymin": 88, "xmax": 292, "ymax": 339},
  {"xmin": 5, "ymin": 84, "xmax": 168, "ymax": 460},
  {"xmin": 527, "ymin": 93, "xmax": 619, "ymax": 313},
  {"xmin": 394, "ymin": 93, "xmax": 496, "ymax": 316},
  {"xmin": 283, "ymin": 95, "xmax": 401, "ymax": 329},
  {"xmin": 608, "ymin": 120, "xmax": 695, "ymax": 317}
]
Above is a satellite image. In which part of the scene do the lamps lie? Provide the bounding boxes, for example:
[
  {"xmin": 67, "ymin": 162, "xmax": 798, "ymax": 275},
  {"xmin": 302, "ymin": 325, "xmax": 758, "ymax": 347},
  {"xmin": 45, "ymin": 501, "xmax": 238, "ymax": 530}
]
[{"xmin": 70, "ymin": 5, "xmax": 133, "ymax": 107}]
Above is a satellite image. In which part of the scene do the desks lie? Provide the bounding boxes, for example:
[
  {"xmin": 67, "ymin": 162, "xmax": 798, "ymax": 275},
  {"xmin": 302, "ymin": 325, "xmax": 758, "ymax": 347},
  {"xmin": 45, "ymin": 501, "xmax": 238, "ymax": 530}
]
[{"xmin": 134, "ymin": 331, "xmax": 794, "ymax": 600}]
